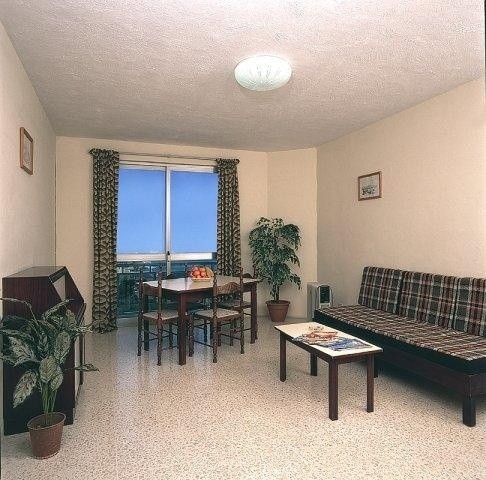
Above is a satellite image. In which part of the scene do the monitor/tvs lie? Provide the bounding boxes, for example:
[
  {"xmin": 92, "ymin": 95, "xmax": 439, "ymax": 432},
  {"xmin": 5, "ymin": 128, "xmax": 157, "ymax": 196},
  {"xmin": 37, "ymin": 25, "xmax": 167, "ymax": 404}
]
[{"xmin": 50, "ymin": 269, "xmax": 66, "ymax": 302}]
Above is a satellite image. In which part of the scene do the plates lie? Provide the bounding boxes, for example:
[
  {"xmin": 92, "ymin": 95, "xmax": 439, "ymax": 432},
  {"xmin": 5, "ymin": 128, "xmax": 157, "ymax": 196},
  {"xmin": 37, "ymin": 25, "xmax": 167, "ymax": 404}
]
[{"xmin": 191, "ymin": 278, "xmax": 211, "ymax": 282}]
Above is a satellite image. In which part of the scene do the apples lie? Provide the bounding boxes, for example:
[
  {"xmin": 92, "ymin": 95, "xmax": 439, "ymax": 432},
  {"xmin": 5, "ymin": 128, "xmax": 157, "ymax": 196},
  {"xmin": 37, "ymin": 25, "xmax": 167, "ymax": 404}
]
[{"xmin": 190, "ymin": 266, "xmax": 208, "ymax": 278}]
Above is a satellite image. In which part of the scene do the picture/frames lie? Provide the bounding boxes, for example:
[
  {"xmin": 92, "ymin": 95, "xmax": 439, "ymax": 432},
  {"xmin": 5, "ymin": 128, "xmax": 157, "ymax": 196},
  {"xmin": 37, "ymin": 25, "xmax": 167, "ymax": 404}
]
[
  {"xmin": 20, "ymin": 127, "xmax": 35, "ymax": 175},
  {"xmin": 358, "ymin": 172, "xmax": 382, "ymax": 199}
]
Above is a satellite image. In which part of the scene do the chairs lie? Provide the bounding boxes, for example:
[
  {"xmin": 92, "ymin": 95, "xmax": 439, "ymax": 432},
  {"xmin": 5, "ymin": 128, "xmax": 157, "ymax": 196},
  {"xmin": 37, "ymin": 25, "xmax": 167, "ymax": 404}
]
[
  {"xmin": 218, "ymin": 270, "xmax": 252, "ymax": 345},
  {"xmin": 189, "ymin": 273, "xmax": 246, "ymax": 363},
  {"xmin": 138, "ymin": 267, "xmax": 187, "ymax": 366}
]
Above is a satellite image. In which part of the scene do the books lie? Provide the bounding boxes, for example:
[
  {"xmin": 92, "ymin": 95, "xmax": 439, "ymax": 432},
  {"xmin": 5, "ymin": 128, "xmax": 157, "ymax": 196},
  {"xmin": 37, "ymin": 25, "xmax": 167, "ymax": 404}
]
[{"xmin": 291, "ymin": 329, "xmax": 372, "ymax": 351}]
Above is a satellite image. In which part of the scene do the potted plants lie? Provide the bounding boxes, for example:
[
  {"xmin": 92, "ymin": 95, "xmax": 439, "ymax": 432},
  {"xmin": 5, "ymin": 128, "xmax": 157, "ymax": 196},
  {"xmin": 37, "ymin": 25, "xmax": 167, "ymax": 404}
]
[
  {"xmin": 248, "ymin": 217, "xmax": 302, "ymax": 323},
  {"xmin": 0, "ymin": 298, "xmax": 100, "ymax": 459}
]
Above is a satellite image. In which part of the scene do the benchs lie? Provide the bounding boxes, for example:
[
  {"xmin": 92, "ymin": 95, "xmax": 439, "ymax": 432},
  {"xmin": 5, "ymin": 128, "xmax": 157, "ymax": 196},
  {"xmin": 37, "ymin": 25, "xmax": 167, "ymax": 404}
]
[{"xmin": 312, "ymin": 264, "xmax": 486, "ymax": 427}]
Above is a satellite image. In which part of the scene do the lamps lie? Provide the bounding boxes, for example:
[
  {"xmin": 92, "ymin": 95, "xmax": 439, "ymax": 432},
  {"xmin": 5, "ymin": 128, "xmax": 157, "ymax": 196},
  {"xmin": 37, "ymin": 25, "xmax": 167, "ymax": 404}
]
[{"xmin": 234, "ymin": 56, "xmax": 291, "ymax": 92}]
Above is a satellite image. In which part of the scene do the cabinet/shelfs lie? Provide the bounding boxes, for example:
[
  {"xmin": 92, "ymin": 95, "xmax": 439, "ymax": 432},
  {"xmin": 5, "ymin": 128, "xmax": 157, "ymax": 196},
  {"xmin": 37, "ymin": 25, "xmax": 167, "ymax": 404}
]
[{"xmin": 1, "ymin": 266, "xmax": 87, "ymax": 436}]
